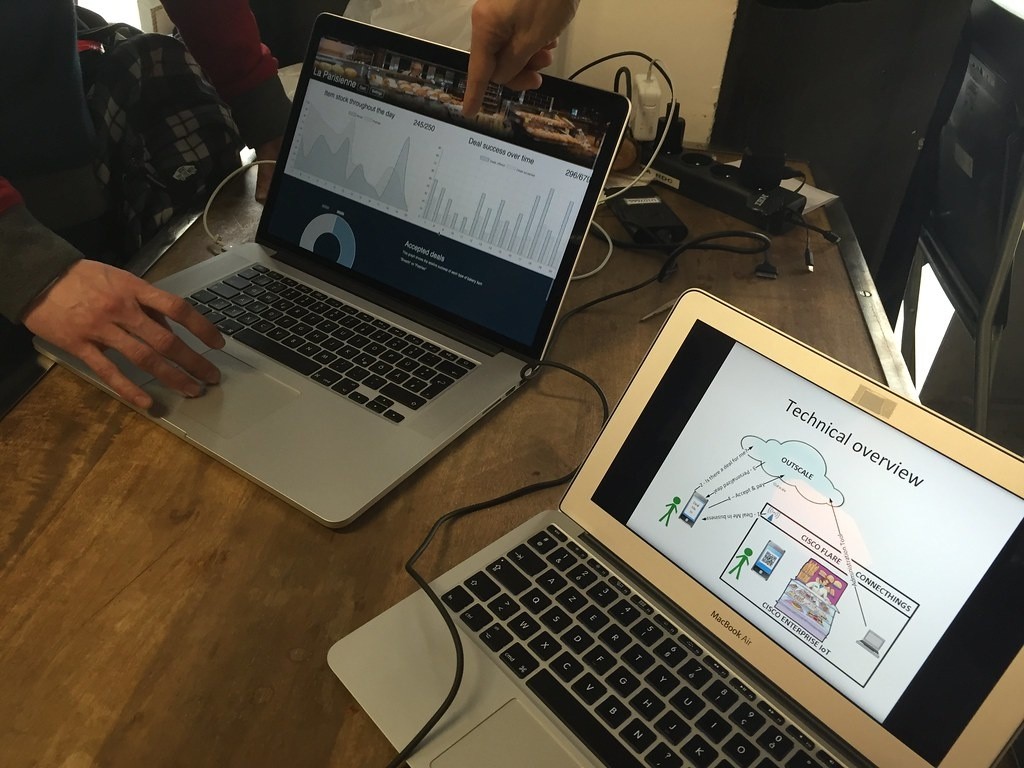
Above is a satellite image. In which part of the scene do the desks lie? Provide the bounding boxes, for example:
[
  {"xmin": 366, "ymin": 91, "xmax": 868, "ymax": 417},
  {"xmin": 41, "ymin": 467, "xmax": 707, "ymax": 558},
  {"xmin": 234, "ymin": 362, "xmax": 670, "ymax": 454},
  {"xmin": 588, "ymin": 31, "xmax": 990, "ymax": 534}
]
[{"xmin": 0, "ymin": 161, "xmax": 919, "ymax": 768}]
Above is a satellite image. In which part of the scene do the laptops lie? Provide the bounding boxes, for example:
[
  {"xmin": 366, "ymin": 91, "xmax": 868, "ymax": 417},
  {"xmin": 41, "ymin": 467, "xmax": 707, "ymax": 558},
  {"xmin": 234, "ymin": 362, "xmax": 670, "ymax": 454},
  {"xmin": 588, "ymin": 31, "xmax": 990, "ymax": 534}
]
[
  {"xmin": 31, "ymin": 12, "xmax": 631, "ymax": 530},
  {"xmin": 325, "ymin": 289, "xmax": 1024, "ymax": 768}
]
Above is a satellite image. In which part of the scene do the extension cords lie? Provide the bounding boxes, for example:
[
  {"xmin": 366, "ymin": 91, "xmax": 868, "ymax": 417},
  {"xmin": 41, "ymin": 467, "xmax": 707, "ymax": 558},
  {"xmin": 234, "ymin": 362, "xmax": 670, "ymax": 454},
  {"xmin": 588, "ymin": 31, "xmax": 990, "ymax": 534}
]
[{"xmin": 621, "ymin": 127, "xmax": 807, "ymax": 234}]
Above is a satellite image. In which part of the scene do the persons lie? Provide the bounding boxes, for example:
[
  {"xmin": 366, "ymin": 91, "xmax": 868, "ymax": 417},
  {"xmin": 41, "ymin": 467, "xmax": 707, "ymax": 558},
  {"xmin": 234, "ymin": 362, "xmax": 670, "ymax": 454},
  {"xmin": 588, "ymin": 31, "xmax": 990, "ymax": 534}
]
[
  {"xmin": 0, "ymin": 0, "xmax": 292, "ymax": 409},
  {"xmin": 462, "ymin": 0, "xmax": 581, "ymax": 116}
]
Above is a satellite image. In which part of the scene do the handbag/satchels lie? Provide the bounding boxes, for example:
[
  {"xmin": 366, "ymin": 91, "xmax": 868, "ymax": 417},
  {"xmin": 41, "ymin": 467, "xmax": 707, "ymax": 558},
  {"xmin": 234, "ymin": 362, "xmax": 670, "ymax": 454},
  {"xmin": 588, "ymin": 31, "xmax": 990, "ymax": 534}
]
[{"xmin": 76, "ymin": 6, "xmax": 245, "ymax": 220}]
